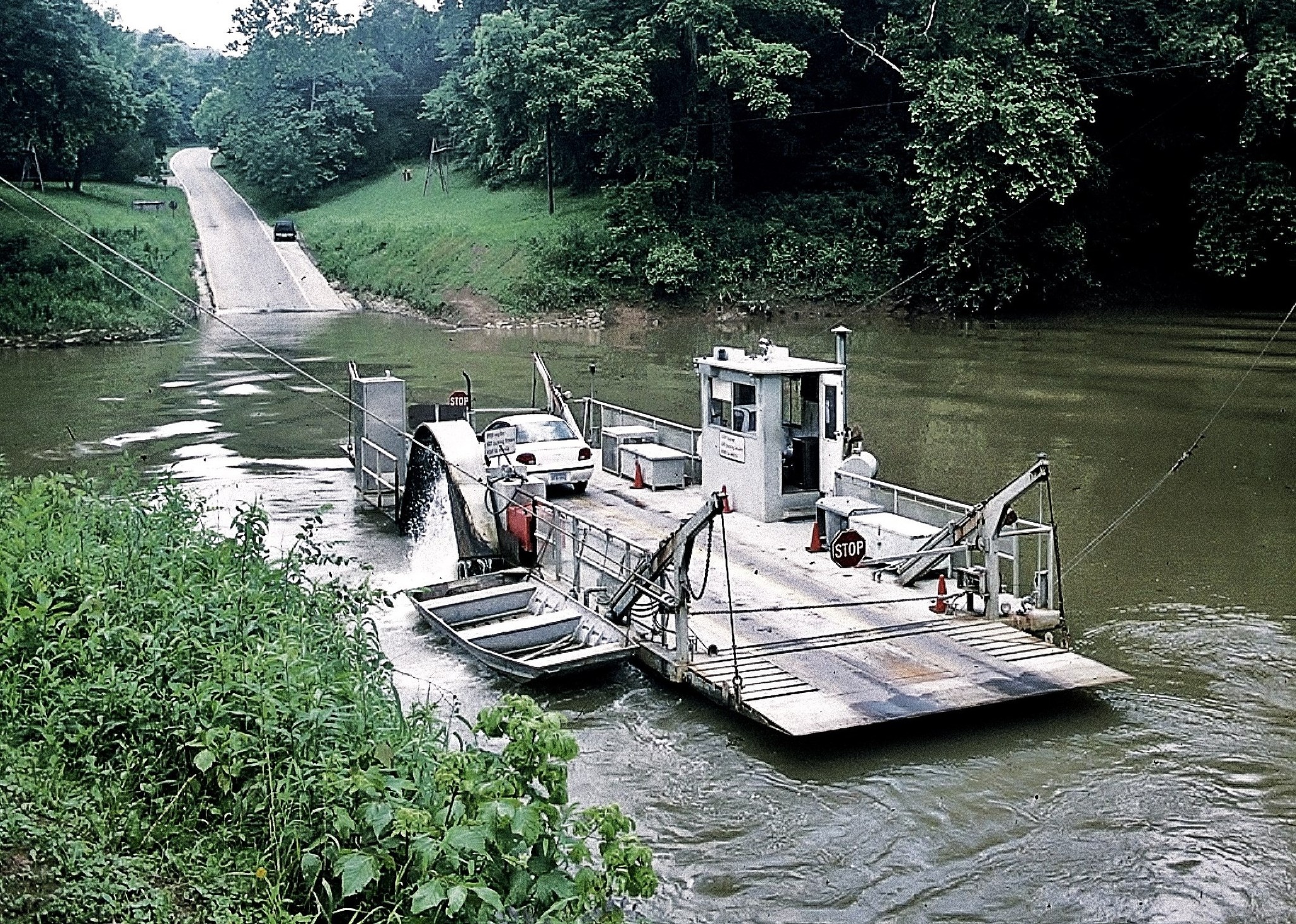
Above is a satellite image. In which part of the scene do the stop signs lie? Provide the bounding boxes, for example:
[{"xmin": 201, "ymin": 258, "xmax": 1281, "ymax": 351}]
[
  {"xmin": 829, "ymin": 529, "xmax": 867, "ymax": 569},
  {"xmin": 448, "ymin": 391, "xmax": 470, "ymax": 412}
]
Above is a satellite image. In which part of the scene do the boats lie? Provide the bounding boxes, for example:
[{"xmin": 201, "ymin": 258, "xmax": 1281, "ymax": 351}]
[{"xmin": 401, "ymin": 570, "xmax": 639, "ymax": 691}]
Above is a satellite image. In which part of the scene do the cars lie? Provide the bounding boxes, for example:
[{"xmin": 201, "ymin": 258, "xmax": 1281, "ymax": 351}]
[
  {"xmin": 272, "ymin": 221, "xmax": 296, "ymax": 242},
  {"xmin": 473, "ymin": 411, "xmax": 593, "ymax": 494}
]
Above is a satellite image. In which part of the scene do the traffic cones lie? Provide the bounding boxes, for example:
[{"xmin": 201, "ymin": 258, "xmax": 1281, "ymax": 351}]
[
  {"xmin": 805, "ymin": 521, "xmax": 830, "ymax": 554},
  {"xmin": 929, "ymin": 574, "xmax": 952, "ymax": 614},
  {"xmin": 719, "ymin": 486, "xmax": 737, "ymax": 516},
  {"xmin": 628, "ymin": 462, "xmax": 645, "ymax": 489}
]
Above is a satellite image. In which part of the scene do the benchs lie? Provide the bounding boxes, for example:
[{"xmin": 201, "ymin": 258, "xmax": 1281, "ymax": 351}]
[
  {"xmin": 456, "ymin": 607, "xmax": 583, "ymax": 651},
  {"xmin": 419, "ymin": 580, "xmax": 535, "ymax": 625}
]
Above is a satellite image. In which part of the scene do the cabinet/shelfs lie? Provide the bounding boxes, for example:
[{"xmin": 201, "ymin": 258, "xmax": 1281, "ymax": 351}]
[
  {"xmin": 815, "ymin": 495, "xmax": 954, "ymax": 578},
  {"xmin": 601, "ymin": 424, "xmax": 689, "ymax": 491}
]
